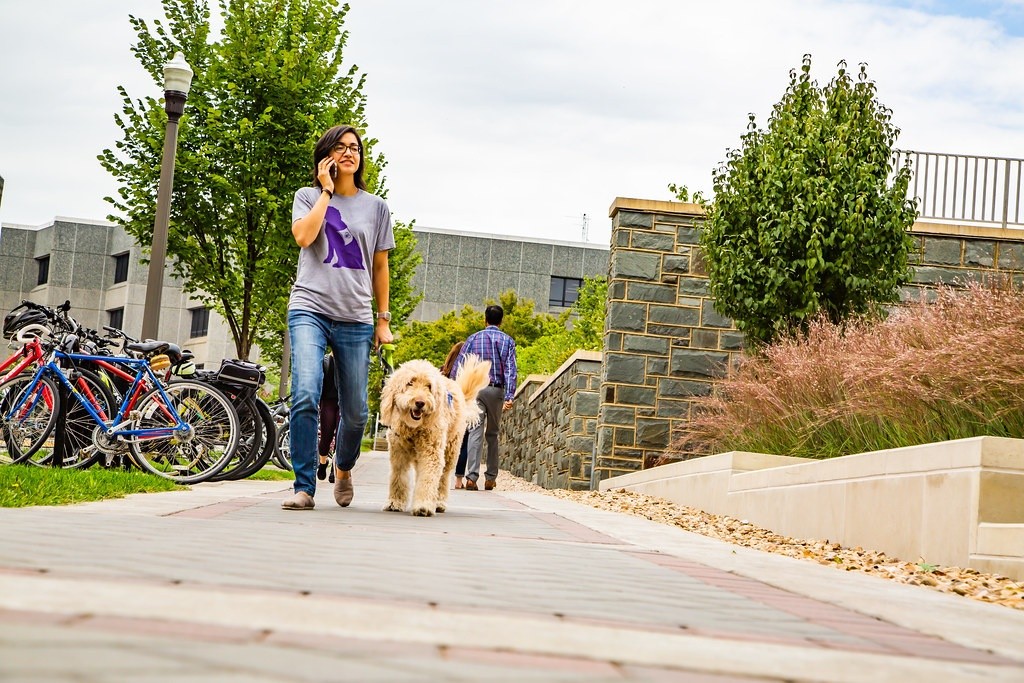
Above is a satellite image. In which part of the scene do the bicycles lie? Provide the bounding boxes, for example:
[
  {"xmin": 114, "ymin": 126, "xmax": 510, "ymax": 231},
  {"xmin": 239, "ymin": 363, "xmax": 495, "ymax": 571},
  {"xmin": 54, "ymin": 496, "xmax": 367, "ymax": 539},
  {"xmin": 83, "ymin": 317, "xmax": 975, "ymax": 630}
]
[{"xmin": 0, "ymin": 299, "xmax": 334, "ymax": 485}]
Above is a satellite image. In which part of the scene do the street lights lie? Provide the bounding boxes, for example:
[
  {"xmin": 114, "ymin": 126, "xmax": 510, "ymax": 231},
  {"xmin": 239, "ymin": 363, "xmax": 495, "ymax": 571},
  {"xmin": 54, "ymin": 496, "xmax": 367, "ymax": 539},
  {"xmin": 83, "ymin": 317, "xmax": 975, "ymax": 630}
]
[{"xmin": 140, "ymin": 50, "xmax": 194, "ymax": 343}]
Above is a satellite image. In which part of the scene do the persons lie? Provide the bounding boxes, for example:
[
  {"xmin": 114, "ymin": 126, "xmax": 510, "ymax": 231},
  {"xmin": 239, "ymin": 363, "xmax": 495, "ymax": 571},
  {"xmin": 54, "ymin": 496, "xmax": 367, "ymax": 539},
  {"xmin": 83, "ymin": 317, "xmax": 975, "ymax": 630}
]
[
  {"xmin": 451, "ymin": 306, "xmax": 518, "ymax": 491},
  {"xmin": 317, "ymin": 350, "xmax": 341, "ymax": 483},
  {"xmin": 281, "ymin": 125, "xmax": 393, "ymax": 510},
  {"xmin": 440, "ymin": 342, "xmax": 469, "ymax": 489}
]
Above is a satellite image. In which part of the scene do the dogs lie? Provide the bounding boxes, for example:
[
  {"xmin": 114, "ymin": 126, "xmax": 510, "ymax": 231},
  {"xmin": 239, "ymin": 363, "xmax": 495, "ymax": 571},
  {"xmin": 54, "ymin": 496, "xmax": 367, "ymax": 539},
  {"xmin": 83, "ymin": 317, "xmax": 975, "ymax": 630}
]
[{"xmin": 379, "ymin": 352, "xmax": 493, "ymax": 516}]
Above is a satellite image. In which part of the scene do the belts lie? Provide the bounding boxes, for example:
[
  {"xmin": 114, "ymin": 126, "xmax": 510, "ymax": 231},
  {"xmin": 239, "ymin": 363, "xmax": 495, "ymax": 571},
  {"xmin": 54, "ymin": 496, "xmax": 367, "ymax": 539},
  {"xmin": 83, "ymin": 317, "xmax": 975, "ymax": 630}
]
[{"xmin": 488, "ymin": 383, "xmax": 504, "ymax": 389}]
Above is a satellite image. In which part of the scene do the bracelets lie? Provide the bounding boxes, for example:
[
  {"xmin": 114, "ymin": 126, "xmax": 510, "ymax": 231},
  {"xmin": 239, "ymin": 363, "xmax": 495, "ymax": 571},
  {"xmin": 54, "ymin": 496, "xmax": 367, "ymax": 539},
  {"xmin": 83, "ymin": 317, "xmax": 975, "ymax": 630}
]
[{"xmin": 321, "ymin": 189, "xmax": 332, "ymax": 199}]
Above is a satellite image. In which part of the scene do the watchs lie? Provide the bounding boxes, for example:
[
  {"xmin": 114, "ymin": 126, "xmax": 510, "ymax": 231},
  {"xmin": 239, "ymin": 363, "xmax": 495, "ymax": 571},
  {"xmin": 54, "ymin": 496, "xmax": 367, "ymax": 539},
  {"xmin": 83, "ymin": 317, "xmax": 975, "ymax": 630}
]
[{"xmin": 377, "ymin": 312, "xmax": 391, "ymax": 321}]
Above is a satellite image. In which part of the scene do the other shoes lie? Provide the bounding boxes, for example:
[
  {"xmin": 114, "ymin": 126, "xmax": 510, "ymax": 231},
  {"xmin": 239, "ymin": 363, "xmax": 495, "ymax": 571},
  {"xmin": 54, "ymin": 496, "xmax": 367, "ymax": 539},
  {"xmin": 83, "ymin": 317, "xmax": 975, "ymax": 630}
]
[
  {"xmin": 485, "ymin": 481, "xmax": 496, "ymax": 490},
  {"xmin": 329, "ymin": 462, "xmax": 334, "ymax": 483},
  {"xmin": 466, "ymin": 480, "xmax": 479, "ymax": 490},
  {"xmin": 332, "ymin": 453, "xmax": 355, "ymax": 507},
  {"xmin": 317, "ymin": 459, "xmax": 328, "ymax": 481},
  {"xmin": 281, "ymin": 493, "xmax": 315, "ymax": 509}
]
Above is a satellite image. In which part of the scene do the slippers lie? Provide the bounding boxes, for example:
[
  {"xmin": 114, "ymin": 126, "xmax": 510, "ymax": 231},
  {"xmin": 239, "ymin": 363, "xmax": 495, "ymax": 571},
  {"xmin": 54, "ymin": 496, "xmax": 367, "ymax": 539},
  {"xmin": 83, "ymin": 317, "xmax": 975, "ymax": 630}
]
[{"xmin": 455, "ymin": 483, "xmax": 464, "ymax": 489}]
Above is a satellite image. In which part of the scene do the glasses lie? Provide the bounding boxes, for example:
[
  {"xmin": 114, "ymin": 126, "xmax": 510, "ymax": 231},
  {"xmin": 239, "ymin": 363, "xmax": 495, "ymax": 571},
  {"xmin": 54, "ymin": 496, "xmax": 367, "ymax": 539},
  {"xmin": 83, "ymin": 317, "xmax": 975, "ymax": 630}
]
[{"xmin": 333, "ymin": 144, "xmax": 361, "ymax": 154}]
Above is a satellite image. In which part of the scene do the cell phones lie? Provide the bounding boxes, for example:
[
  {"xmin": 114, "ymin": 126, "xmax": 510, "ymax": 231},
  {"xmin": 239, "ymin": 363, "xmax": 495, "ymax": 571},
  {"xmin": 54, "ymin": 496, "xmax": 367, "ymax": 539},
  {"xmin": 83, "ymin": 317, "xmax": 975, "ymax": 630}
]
[{"xmin": 330, "ymin": 163, "xmax": 337, "ymax": 179}]
